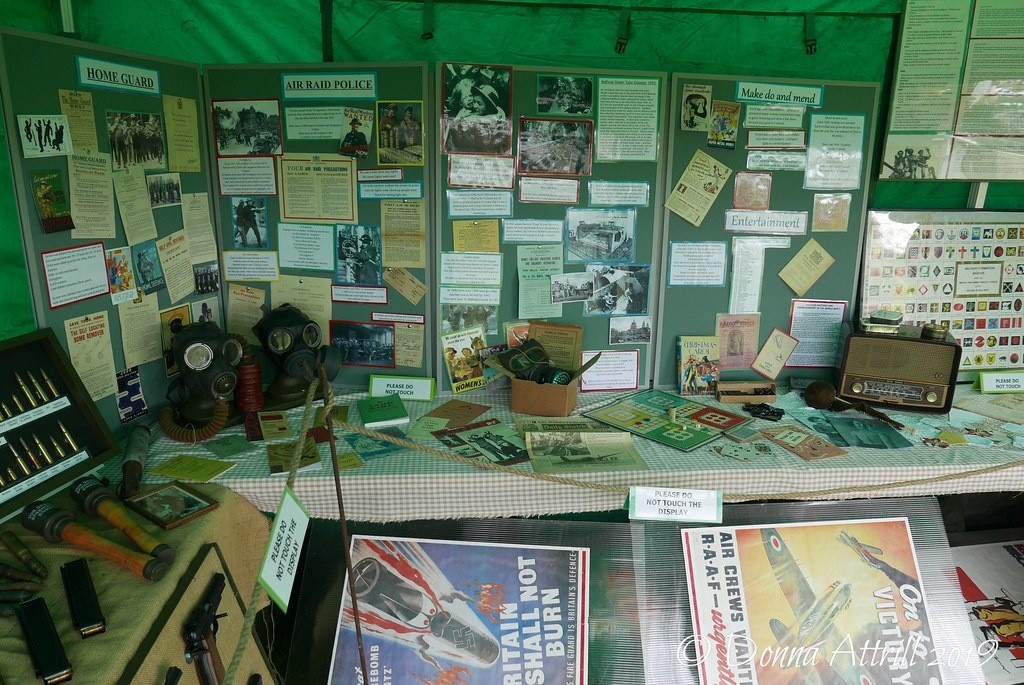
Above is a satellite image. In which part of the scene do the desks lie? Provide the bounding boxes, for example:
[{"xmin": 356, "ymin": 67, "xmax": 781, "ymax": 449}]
[{"xmin": 106, "ymin": 384, "xmax": 1024, "ymax": 524}]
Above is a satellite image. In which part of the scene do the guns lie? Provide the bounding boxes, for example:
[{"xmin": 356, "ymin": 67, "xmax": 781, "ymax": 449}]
[{"xmin": 180, "ymin": 572, "xmax": 230, "ymax": 685}]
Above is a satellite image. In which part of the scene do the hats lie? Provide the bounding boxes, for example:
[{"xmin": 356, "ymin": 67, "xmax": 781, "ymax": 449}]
[
  {"xmin": 360, "ymin": 235, "xmax": 372, "ymax": 242},
  {"xmin": 443, "ymin": 347, "xmax": 457, "ymax": 353},
  {"xmin": 245, "ymin": 198, "xmax": 252, "ymax": 202},
  {"xmin": 40, "ymin": 180, "xmax": 47, "ymax": 185},
  {"xmin": 240, "ymin": 199, "xmax": 244, "ymax": 203},
  {"xmin": 349, "ymin": 118, "xmax": 362, "ymax": 126},
  {"xmin": 461, "ymin": 348, "xmax": 472, "ymax": 354},
  {"xmin": 471, "ymin": 85, "xmax": 500, "ymax": 114}
]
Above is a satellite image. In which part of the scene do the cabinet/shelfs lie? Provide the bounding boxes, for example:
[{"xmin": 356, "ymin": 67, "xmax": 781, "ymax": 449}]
[{"xmin": 0, "ymin": 482, "xmax": 280, "ymax": 685}]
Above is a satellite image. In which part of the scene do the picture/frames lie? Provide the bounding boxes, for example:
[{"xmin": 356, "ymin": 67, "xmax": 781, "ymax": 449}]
[{"xmin": 123, "ymin": 480, "xmax": 220, "ymax": 530}]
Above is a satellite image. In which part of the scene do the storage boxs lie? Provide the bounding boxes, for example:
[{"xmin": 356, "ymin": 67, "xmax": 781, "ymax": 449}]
[{"xmin": 482, "ymin": 321, "xmax": 602, "ymax": 417}]
